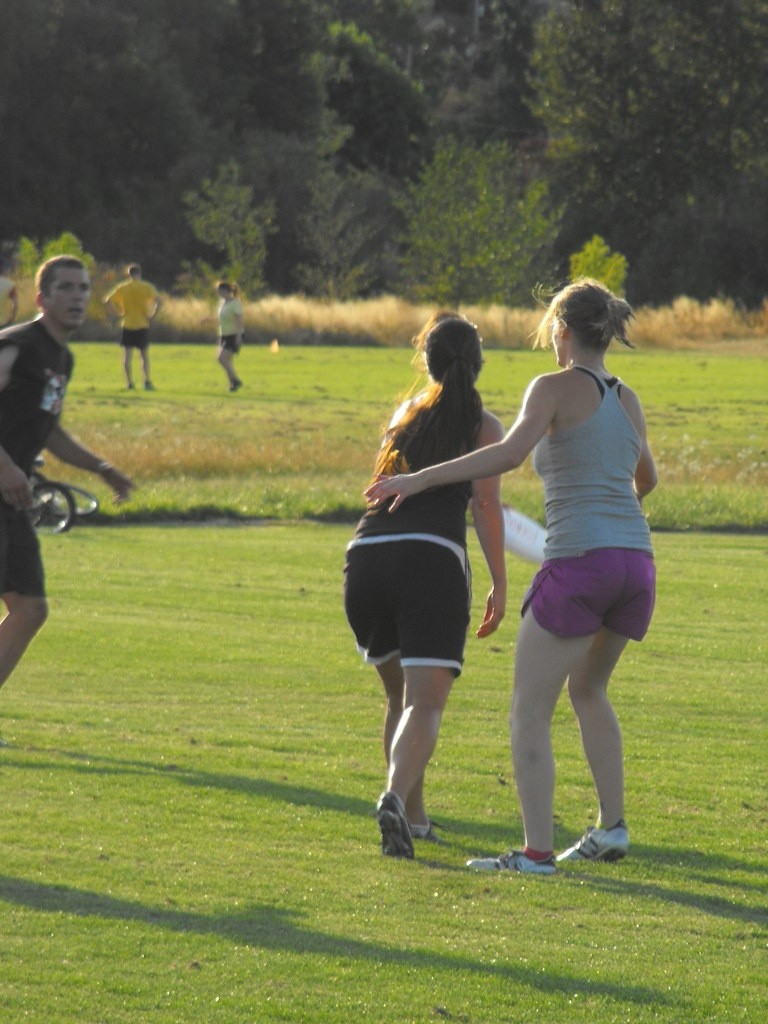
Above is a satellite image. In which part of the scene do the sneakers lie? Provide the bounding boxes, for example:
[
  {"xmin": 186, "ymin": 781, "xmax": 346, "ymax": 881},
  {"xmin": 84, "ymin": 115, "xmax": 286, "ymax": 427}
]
[
  {"xmin": 410, "ymin": 819, "xmax": 448, "ymax": 845},
  {"xmin": 466, "ymin": 849, "xmax": 557, "ymax": 875},
  {"xmin": 376, "ymin": 791, "xmax": 415, "ymax": 860},
  {"xmin": 556, "ymin": 818, "xmax": 629, "ymax": 862}
]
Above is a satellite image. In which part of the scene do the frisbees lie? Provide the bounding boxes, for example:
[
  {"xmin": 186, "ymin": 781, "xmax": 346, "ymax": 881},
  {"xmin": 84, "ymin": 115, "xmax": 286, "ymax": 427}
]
[{"xmin": 498, "ymin": 507, "xmax": 548, "ymax": 566}]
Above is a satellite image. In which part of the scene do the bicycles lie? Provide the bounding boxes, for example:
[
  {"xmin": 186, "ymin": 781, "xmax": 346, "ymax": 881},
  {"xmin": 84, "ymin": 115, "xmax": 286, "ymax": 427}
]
[{"xmin": 28, "ymin": 457, "xmax": 100, "ymax": 533}]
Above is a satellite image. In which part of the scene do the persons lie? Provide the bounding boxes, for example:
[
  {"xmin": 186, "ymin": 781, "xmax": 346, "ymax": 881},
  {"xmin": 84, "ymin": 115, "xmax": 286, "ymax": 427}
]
[
  {"xmin": 0, "ymin": 241, "xmax": 20, "ymax": 328},
  {"xmin": 215, "ymin": 281, "xmax": 247, "ymax": 392},
  {"xmin": 0, "ymin": 253, "xmax": 138, "ymax": 689},
  {"xmin": 365, "ymin": 275, "xmax": 658, "ymax": 876},
  {"xmin": 343, "ymin": 312, "xmax": 510, "ymax": 858},
  {"xmin": 104, "ymin": 262, "xmax": 163, "ymax": 392}
]
